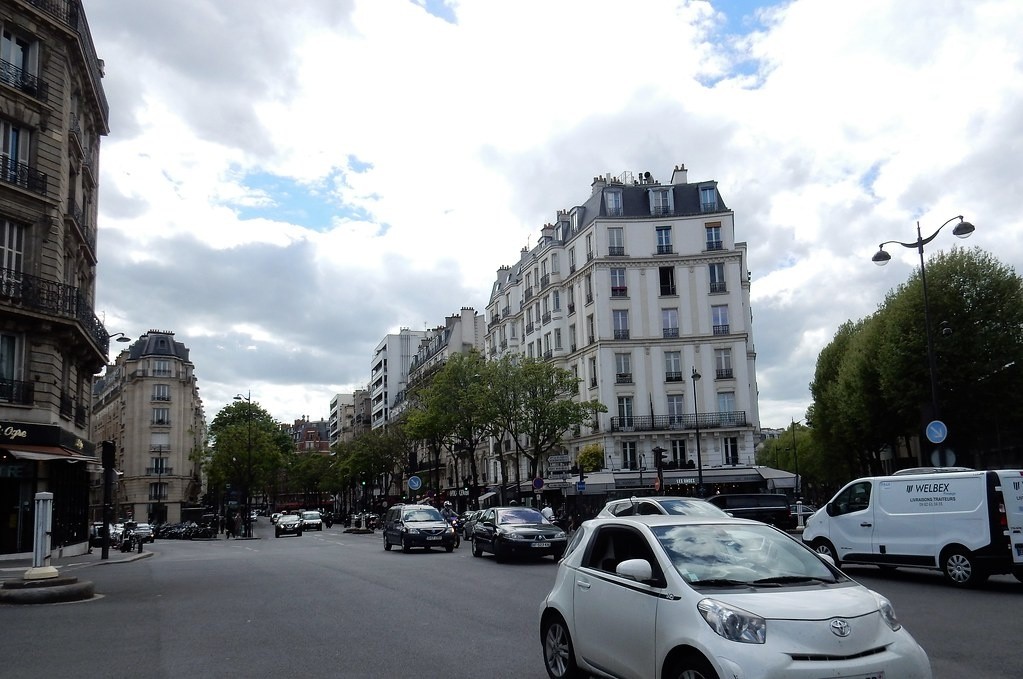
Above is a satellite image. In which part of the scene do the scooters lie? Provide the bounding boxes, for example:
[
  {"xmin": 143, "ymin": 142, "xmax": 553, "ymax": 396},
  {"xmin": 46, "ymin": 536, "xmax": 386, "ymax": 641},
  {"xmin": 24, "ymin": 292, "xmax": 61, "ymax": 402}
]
[
  {"xmin": 547, "ymin": 514, "xmax": 560, "ymax": 528},
  {"xmin": 148, "ymin": 521, "xmax": 219, "ymax": 540}
]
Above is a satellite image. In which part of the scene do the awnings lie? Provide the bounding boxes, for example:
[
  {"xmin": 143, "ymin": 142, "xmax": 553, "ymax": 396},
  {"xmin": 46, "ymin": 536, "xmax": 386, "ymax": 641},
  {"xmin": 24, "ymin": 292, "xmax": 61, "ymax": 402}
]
[
  {"xmin": 478, "ymin": 492, "xmax": 496, "ymax": 501},
  {"xmin": 0, "ymin": 444, "xmax": 102, "ymax": 464}
]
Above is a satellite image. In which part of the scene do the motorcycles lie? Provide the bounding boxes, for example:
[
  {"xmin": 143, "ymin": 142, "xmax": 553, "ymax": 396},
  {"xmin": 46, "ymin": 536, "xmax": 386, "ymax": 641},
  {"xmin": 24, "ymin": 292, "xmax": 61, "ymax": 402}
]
[{"xmin": 444, "ymin": 515, "xmax": 463, "ymax": 549}]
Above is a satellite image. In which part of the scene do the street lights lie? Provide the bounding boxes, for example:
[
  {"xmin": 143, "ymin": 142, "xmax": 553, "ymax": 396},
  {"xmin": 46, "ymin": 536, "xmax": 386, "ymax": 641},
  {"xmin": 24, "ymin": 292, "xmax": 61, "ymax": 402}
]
[
  {"xmin": 233, "ymin": 392, "xmax": 252, "ymax": 538},
  {"xmin": 691, "ymin": 366, "xmax": 702, "ymax": 484},
  {"xmin": 872, "ymin": 215, "xmax": 976, "ymax": 465},
  {"xmin": 776, "ymin": 445, "xmax": 790, "ymax": 469},
  {"xmin": 792, "ymin": 419, "xmax": 801, "ymax": 500}
]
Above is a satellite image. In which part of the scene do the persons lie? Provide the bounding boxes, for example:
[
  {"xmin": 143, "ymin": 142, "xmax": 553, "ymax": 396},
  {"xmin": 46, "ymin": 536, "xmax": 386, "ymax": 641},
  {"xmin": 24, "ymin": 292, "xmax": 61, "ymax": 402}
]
[
  {"xmin": 440, "ymin": 501, "xmax": 460, "ymax": 525},
  {"xmin": 324, "ymin": 507, "xmax": 373, "ymax": 527},
  {"xmin": 540, "ymin": 502, "xmax": 604, "ymax": 534},
  {"xmin": 219, "ymin": 508, "xmax": 241, "ymax": 539}
]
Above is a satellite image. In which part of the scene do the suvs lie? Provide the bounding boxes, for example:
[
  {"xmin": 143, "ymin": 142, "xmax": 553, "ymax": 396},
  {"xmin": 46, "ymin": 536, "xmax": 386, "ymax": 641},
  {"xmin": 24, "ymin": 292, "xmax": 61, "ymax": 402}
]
[
  {"xmin": 134, "ymin": 522, "xmax": 154, "ymax": 543},
  {"xmin": 89, "ymin": 521, "xmax": 133, "ymax": 547},
  {"xmin": 383, "ymin": 503, "xmax": 456, "ymax": 554}
]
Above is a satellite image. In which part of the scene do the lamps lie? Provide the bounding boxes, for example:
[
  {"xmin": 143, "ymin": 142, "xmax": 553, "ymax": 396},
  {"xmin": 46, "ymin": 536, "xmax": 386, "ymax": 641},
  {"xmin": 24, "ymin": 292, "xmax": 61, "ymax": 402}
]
[{"xmin": 109, "ymin": 333, "xmax": 131, "ymax": 342}]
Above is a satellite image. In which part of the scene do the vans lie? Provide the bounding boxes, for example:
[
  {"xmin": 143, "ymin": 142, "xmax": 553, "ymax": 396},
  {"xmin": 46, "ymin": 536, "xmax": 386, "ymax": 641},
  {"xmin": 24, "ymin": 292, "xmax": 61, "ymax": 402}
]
[
  {"xmin": 801, "ymin": 465, "xmax": 1023, "ymax": 589},
  {"xmin": 705, "ymin": 493, "xmax": 790, "ymax": 533}
]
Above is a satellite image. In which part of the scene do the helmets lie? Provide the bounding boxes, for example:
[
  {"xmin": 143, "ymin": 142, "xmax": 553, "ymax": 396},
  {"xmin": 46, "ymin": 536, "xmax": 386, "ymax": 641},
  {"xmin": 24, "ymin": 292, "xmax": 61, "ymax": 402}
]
[
  {"xmin": 444, "ymin": 500, "xmax": 453, "ymax": 511},
  {"xmin": 542, "ymin": 502, "xmax": 548, "ymax": 507}
]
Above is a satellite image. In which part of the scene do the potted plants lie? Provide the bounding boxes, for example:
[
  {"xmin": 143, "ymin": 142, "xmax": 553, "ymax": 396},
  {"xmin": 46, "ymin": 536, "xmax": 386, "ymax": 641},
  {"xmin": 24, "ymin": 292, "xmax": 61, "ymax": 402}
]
[
  {"xmin": 687, "ymin": 460, "xmax": 695, "ymax": 469},
  {"xmin": 667, "ymin": 461, "xmax": 676, "ymax": 469}
]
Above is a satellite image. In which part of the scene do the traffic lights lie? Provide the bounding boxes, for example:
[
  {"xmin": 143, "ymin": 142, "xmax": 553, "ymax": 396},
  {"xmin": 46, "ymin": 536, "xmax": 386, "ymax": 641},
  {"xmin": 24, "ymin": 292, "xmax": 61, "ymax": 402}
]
[{"xmin": 653, "ymin": 446, "xmax": 668, "ymax": 468}]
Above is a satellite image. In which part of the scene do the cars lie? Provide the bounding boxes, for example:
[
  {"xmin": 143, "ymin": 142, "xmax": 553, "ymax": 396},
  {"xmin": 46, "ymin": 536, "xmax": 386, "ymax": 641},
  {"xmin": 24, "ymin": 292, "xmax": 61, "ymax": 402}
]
[
  {"xmin": 471, "ymin": 507, "xmax": 568, "ymax": 564},
  {"xmin": 456, "ymin": 510, "xmax": 476, "ymax": 534},
  {"xmin": 537, "ymin": 515, "xmax": 933, "ymax": 679},
  {"xmin": 275, "ymin": 514, "xmax": 303, "ymax": 538},
  {"xmin": 248, "ymin": 507, "xmax": 386, "ymax": 531},
  {"xmin": 593, "ymin": 496, "xmax": 734, "ymax": 517},
  {"xmin": 461, "ymin": 509, "xmax": 494, "ymax": 541},
  {"xmin": 789, "ymin": 504, "xmax": 816, "ymax": 530}
]
